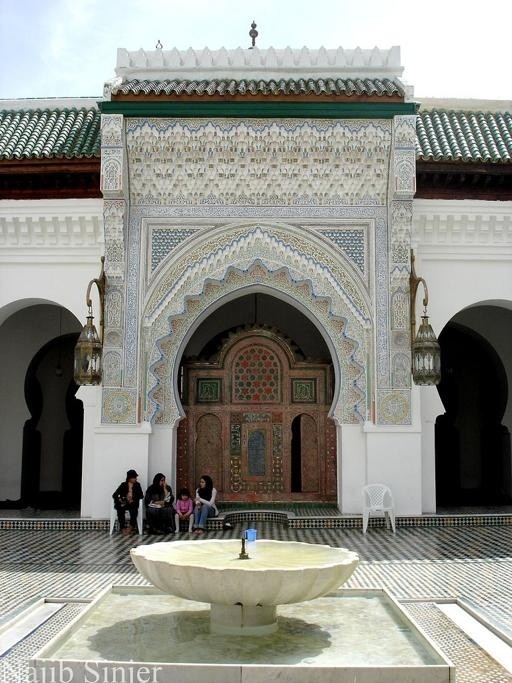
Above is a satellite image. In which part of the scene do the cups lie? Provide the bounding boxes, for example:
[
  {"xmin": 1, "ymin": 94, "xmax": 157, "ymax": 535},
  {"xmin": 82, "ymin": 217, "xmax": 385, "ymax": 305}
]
[{"xmin": 244, "ymin": 528, "xmax": 258, "ymax": 541}]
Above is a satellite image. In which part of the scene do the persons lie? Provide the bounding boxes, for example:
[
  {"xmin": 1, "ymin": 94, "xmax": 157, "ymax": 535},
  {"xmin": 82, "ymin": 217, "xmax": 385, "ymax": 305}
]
[
  {"xmin": 194, "ymin": 475, "xmax": 219, "ymax": 533},
  {"xmin": 113, "ymin": 469, "xmax": 143, "ymax": 533},
  {"xmin": 144, "ymin": 473, "xmax": 174, "ymax": 535},
  {"xmin": 175, "ymin": 488, "xmax": 194, "ymax": 533}
]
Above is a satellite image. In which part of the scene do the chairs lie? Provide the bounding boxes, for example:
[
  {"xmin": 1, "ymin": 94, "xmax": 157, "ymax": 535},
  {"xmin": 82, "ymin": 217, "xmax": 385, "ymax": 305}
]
[{"xmin": 360, "ymin": 483, "xmax": 398, "ymax": 534}]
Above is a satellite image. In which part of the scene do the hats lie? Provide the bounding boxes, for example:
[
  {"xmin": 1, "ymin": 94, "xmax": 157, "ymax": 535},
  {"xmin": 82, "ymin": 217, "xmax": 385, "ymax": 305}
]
[
  {"xmin": 127, "ymin": 470, "xmax": 138, "ymax": 478},
  {"xmin": 224, "ymin": 523, "xmax": 233, "ymax": 530},
  {"xmin": 180, "ymin": 488, "xmax": 189, "ymax": 496}
]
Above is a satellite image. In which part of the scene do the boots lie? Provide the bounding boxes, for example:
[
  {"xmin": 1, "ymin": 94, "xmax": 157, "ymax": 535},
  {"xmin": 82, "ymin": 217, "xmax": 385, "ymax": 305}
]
[
  {"xmin": 175, "ymin": 514, "xmax": 179, "ymax": 533},
  {"xmin": 189, "ymin": 514, "xmax": 194, "ymax": 533}
]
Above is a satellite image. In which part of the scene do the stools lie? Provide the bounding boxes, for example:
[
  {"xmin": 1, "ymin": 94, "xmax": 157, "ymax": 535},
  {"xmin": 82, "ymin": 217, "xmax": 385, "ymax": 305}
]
[{"xmin": 110, "ymin": 497, "xmax": 145, "ymax": 536}]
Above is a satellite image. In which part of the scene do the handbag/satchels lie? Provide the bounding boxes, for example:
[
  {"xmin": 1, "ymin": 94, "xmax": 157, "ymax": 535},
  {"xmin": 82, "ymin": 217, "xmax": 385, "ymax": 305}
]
[
  {"xmin": 148, "ymin": 504, "xmax": 165, "ymax": 509},
  {"xmin": 118, "ymin": 498, "xmax": 127, "ymax": 507}
]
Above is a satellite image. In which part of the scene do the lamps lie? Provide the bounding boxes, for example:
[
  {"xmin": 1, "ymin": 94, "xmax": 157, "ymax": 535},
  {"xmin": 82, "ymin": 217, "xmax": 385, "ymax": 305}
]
[
  {"xmin": 410, "ymin": 248, "xmax": 444, "ymax": 387},
  {"xmin": 74, "ymin": 254, "xmax": 109, "ymax": 385}
]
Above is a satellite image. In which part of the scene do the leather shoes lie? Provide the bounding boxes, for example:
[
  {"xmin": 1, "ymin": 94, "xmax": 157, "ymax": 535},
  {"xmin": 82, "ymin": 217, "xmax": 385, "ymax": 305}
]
[{"xmin": 194, "ymin": 529, "xmax": 207, "ymax": 537}]
